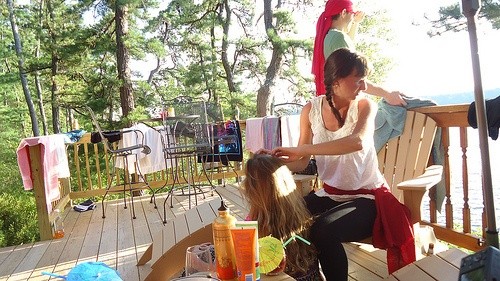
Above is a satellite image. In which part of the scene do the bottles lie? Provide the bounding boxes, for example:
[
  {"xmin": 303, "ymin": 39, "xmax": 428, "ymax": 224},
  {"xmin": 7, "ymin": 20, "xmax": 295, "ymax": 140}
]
[{"xmin": 212, "ymin": 200, "xmax": 238, "ymax": 281}]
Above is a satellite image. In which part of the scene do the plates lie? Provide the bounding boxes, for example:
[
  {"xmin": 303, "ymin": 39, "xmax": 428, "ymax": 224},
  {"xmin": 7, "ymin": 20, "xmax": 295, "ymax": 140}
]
[{"xmin": 169, "ymin": 276, "xmax": 221, "ymax": 281}]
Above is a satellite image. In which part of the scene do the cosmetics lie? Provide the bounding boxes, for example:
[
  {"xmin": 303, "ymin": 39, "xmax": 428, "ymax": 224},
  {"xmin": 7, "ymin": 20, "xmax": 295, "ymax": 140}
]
[{"xmin": 212, "ymin": 200, "xmax": 260, "ymax": 281}]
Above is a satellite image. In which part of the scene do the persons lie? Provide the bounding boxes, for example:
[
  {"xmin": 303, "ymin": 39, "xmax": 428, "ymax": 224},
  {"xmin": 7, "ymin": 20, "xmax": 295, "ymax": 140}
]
[
  {"xmin": 240, "ymin": 153, "xmax": 324, "ymax": 281},
  {"xmin": 311, "ymin": 0, "xmax": 408, "ymax": 107},
  {"xmin": 255, "ymin": 49, "xmax": 392, "ymax": 281}
]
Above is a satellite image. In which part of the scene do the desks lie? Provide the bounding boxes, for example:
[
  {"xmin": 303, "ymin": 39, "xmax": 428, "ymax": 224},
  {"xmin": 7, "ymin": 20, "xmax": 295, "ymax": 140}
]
[{"xmin": 139, "ymin": 114, "xmax": 216, "ymax": 209}]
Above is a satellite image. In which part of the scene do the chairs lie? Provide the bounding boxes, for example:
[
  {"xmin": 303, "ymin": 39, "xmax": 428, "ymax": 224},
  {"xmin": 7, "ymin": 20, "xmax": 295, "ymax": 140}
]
[
  {"xmin": 296, "ymin": 108, "xmax": 444, "ymax": 267},
  {"xmin": 85, "ymin": 95, "xmax": 238, "ymax": 224}
]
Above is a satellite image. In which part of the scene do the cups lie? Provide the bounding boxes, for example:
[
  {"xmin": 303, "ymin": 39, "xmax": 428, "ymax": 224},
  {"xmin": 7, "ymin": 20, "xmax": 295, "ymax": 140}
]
[{"xmin": 185, "ymin": 245, "xmax": 212, "ymax": 277}]
